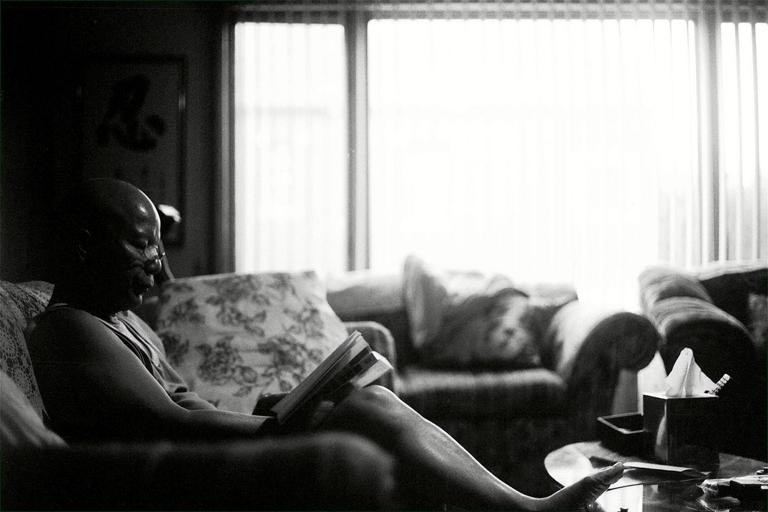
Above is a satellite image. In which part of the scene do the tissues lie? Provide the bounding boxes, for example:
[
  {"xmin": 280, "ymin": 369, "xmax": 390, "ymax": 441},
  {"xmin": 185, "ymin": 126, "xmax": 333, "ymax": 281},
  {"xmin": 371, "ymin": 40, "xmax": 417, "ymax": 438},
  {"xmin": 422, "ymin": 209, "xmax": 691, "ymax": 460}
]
[{"xmin": 637, "ymin": 346, "xmax": 722, "ymax": 470}]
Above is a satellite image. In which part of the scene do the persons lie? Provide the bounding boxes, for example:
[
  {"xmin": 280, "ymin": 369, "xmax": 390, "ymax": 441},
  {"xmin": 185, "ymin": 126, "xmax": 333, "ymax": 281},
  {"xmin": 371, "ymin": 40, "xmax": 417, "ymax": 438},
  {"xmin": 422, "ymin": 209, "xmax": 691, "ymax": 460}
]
[{"xmin": 29, "ymin": 177, "xmax": 625, "ymax": 512}]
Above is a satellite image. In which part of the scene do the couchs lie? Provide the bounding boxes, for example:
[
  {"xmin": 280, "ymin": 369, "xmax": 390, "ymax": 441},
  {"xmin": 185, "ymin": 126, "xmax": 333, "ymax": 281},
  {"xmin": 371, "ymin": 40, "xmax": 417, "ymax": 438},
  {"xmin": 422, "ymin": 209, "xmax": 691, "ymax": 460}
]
[{"xmin": 0, "ymin": 256, "xmax": 766, "ymax": 511}]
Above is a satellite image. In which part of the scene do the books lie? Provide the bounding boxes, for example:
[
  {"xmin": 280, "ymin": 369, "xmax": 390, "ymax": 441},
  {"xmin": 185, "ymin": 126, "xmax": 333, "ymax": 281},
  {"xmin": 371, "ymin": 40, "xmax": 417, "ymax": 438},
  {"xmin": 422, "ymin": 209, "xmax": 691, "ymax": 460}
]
[{"xmin": 269, "ymin": 328, "xmax": 396, "ymax": 425}]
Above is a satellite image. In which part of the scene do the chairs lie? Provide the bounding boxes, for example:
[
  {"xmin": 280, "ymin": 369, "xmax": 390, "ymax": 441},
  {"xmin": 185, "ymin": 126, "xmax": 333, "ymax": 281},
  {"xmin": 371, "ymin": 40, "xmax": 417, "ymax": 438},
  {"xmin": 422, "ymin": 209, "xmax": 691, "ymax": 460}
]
[{"xmin": 631, "ymin": 257, "xmax": 767, "ymax": 413}]
